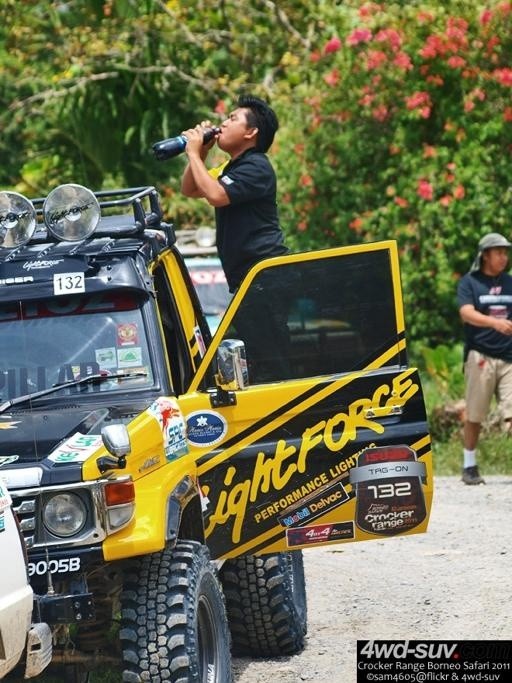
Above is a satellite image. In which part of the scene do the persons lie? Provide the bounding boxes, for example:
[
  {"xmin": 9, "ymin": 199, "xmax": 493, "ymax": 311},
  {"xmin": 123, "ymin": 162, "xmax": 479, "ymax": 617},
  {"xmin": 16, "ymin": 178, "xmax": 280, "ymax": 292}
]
[
  {"xmin": 458, "ymin": 230, "xmax": 512, "ymax": 485},
  {"xmin": 177, "ymin": 89, "xmax": 293, "ymax": 391}
]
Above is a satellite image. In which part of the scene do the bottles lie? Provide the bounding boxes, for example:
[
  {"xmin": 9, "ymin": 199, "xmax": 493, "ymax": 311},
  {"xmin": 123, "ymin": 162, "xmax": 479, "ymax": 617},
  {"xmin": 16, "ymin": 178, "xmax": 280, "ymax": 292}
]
[{"xmin": 150, "ymin": 125, "xmax": 220, "ymax": 161}]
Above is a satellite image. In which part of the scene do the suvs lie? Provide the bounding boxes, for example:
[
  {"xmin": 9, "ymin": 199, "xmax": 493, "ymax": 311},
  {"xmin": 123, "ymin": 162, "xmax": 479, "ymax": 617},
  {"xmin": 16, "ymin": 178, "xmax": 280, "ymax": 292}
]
[
  {"xmin": 0, "ymin": 183, "xmax": 434, "ymax": 682},
  {"xmin": 0, "ymin": 479, "xmax": 55, "ymax": 681},
  {"xmin": 173, "ymin": 225, "xmax": 363, "ymax": 387}
]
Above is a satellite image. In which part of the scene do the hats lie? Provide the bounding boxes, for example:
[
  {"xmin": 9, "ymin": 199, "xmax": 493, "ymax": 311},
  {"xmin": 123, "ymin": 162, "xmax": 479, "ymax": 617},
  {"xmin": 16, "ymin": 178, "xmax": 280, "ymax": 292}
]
[{"xmin": 470, "ymin": 233, "xmax": 512, "ymax": 272}]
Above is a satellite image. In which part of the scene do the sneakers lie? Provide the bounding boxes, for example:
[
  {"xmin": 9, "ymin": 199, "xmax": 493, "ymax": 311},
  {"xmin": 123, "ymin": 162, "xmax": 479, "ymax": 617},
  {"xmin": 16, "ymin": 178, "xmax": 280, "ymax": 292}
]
[{"xmin": 462, "ymin": 465, "xmax": 482, "ymax": 484}]
